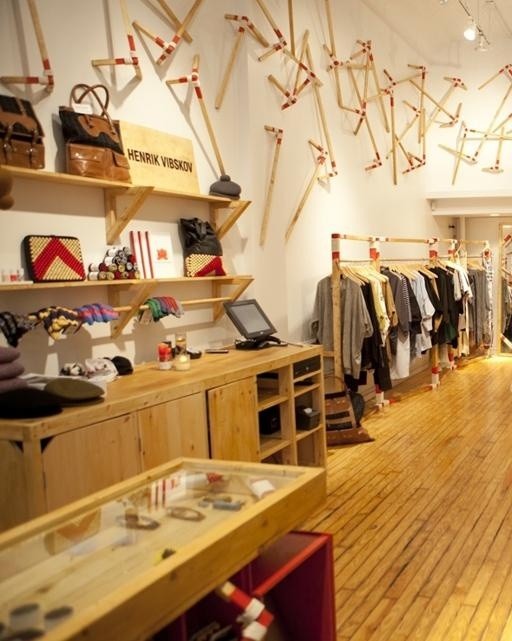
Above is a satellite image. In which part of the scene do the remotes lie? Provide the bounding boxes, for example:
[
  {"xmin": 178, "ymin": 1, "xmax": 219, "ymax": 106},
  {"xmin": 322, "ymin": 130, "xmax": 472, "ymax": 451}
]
[{"xmin": 205, "ymin": 347, "xmax": 230, "ymax": 356}]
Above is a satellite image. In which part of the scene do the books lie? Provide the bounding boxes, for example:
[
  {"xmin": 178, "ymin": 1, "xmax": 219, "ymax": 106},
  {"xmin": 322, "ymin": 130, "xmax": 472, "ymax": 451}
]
[{"xmin": 130, "ymin": 231, "xmax": 178, "ymax": 279}]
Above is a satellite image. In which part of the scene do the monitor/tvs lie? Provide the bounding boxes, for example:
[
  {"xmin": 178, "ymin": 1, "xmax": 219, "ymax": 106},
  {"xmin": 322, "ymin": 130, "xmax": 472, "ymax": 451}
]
[{"xmin": 223, "ymin": 299, "xmax": 291, "ymax": 351}]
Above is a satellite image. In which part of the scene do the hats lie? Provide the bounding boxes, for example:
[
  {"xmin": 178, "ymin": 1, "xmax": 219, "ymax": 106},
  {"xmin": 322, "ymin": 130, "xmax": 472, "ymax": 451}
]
[{"xmin": 209, "ymin": 173, "xmax": 241, "ymax": 199}]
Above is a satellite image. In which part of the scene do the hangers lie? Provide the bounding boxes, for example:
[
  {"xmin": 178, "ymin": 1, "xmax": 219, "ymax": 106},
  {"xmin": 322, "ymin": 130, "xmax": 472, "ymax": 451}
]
[{"xmin": 341, "ymin": 251, "xmax": 494, "ymax": 285}]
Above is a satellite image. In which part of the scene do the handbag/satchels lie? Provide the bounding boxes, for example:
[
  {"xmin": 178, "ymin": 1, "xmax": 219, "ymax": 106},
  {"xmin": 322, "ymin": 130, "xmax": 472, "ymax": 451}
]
[
  {"xmin": 186, "ymin": 254, "xmax": 227, "ymax": 277},
  {"xmin": 0, "ymin": 93, "xmax": 45, "ymax": 169},
  {"xmin": 179, "ymin": 217, "xmax": 224, "ymax": 256},
  {"xmin": 25, "ymin": 236, "xmax": 86, "ymax": 281},
  {"xmin": 58, "ymin": 83, "xmax": 132, "ymax": 186},
  {"xmin": 324, "ymin": 374, "xmax": 357, "ymax": 431}
]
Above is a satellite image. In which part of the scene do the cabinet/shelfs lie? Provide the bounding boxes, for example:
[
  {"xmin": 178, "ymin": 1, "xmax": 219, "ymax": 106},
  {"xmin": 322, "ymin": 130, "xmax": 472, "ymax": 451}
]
[
  {"xmin": 0, "ymin": 389, "xmax": 209, "ymax": 525},
  {"xmin": 257, "ymin": 345, "xmax": 326, "ymax": 475},
  {"xmin": 0, "ymin": 455, "xmax": 337, "ymax": 641},
  {"xmin": 211, "ymin": 376, "xmax": 259, "ymax": 465}
]
